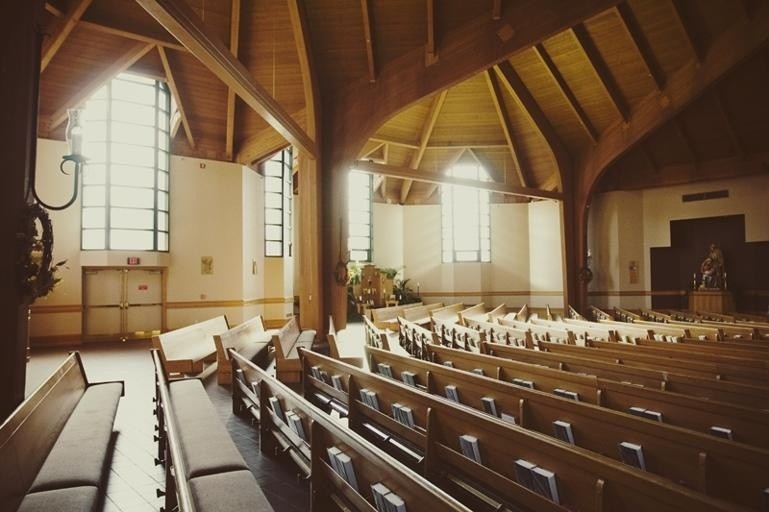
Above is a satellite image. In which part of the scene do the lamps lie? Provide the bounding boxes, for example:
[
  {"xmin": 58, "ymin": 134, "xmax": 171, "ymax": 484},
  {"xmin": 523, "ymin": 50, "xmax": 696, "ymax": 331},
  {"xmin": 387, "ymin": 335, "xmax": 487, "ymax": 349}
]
[{"xmin": 34, "ymin": 107, "xmax": 88, "ymax": 210}]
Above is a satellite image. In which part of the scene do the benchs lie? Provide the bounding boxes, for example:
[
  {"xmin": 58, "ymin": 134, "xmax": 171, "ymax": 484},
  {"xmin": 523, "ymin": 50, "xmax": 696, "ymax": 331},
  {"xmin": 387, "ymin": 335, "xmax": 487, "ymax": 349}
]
[{"xmin": 0, "ymin": 301, "xmax": 769, "ymax": 512}]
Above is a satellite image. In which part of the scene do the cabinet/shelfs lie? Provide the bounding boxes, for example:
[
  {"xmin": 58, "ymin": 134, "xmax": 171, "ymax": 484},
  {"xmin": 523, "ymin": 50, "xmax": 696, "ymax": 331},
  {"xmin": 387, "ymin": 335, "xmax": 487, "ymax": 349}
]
[{"xmin": 361, "ymin": 264, "xmax": 394, "ymax": 308}]
